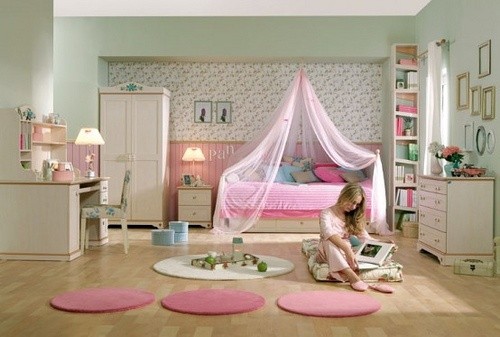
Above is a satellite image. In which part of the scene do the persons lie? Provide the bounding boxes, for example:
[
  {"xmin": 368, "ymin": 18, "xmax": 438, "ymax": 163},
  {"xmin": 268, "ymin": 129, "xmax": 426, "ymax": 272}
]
[
  {"xmin": 315, "ymin": 183, "xmax": 399, "ymax": 291},
  {"xmin": 199, "ymin": 108, "xmax": 205, "ymax": 122},
  {"xmin": 221, "ymin": 108, "xmax": 226, "ymax": 122}
]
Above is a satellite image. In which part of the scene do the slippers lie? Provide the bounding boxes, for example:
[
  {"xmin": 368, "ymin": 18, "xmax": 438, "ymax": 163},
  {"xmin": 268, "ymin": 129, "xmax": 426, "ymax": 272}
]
[
  {"xmin": 369, "ymin": 283, "xmax": 395, "ymax": 294},
  {"xmin": 351, "ymin": 281, "xmax": 367, "ymax": 291}
]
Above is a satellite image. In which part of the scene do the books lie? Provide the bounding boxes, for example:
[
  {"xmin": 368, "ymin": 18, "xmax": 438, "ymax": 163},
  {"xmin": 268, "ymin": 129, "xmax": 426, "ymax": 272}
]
[
  {"xmin": 397, "ymin": 105, "xmax": 416, "ymax": 113},
  {"xmin": 395, "ymin": 165, "xmax": 405, "ymax": 183},
  {"xmin": 407, "ymin": 143, "xmax": 419, "ymax": 161},
  {"xmin": 400, "ymin": 213, "xmax": 415, "ymax": 231},
  {"xmin": 405, "ymin": 71, "xmax": 419, "ymax": 90},
  {"xmin": 395, "ymin": 116, "xmax": 404, "ymax": 136},
  {"xmin": 395, "ymin": 188, "xmax": 417, "ymax": 208}
]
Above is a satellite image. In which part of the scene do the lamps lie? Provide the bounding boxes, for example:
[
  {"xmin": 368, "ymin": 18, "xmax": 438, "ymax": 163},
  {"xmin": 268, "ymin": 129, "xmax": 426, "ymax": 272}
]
[
  {"xmin": 181, "ymin": 146, "xmax": 206, "ymax": 186},
  {"xmin": 74, "ymin": 127, "xmax": 105, "ymax": 178}
]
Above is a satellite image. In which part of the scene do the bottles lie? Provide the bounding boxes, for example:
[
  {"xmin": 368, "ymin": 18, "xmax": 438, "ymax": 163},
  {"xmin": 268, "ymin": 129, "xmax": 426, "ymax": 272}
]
[{"xmin": 233, "ymin": 230, "xmax": 242, "ymax": 264}]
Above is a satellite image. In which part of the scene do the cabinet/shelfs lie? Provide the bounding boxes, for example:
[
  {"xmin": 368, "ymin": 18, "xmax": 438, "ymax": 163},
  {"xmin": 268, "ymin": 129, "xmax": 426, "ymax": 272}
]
[
  {"xmin": 99, "ymin": 81, "xmax": 170, "ymax": 229},
  {"xmin": 0, "ymin": 108, "xmax": 110, "ymax": 262},
  {"xmin": 417, "ymin": 173, "xmax": 495, "ymax": 267},
  {"xmin": 382, "ymin": 42, "xmax": 420, "ymax": 233}
]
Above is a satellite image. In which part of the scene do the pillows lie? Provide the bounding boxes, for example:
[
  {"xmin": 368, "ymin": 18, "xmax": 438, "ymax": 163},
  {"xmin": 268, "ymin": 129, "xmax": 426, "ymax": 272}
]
[{"xmin": 246, "ymin": 156, "xmax": 367, "ymax": 185}]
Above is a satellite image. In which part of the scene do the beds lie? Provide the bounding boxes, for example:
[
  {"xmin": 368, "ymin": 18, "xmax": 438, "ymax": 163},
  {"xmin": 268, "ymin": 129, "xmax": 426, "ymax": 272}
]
[{"xmin": 221, "ymin": 177, "xmax": 380, "ymax": 234}]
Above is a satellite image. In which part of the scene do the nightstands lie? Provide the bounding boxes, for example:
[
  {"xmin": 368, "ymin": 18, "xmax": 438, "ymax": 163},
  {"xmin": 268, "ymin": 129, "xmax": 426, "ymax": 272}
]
[{"xmin": 176, "ymin": 184, "xmax": 216, "ymax": 229}]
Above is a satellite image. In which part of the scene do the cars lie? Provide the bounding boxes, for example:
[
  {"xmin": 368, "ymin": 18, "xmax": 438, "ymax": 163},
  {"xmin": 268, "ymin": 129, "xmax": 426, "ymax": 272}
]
[{"xmin": 450, "ymin": 163, "xmax": 487, "ymax": 177}]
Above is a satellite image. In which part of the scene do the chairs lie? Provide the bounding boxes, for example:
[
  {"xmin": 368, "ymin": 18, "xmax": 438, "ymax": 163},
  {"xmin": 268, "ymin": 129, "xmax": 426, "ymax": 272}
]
[{"xmin": 80, "ymin": 171, "xmax": 132, "ymax": 255}]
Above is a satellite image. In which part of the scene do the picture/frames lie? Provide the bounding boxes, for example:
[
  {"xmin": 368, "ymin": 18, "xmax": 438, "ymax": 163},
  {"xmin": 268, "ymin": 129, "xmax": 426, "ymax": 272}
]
[
  {"xmin": 469, "ymin": 86, "xmax": 481, "ymax": 115},
  {"xmin": 194, "ymin": 101, "xmax": 212, "ymax": 123},
  {"xmin": 456, "ymin": 73, "xmax": 469, "ymax": 110},
  {"xmin": 477, "ymin": 39, "xmax": 490, "ymax": 78},
  {"xmin": 463, "ymin": 122, "xmax": 475, "ymax": 153},
  {"xmin": 216, "ymin": 101, "xmax": 232, "ymax": 124},
  {"xmin": 485, "ymin": 130, "xmax": 496, "ymax": 154},
  {"xmin": 476, "ymin": 126, "xmax": 486, "ymax": 155},
  {"xmin": 481, "ymin": 85, "xmax": 494, "ymax": 120}
]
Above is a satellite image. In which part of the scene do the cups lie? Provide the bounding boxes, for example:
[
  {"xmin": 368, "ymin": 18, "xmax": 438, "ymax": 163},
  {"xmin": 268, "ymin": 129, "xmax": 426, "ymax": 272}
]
[{"xmin": 43, "ymin": 167, "xmax": 52, "ymax": 181}]
